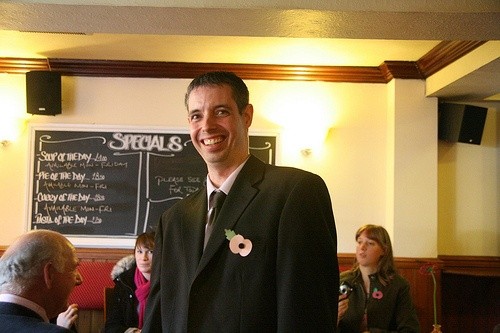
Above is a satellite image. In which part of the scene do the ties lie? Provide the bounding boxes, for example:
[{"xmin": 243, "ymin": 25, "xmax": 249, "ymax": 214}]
[{"xmin": 203, "ymin": 191, "xmax": 226, "ymax": 255}]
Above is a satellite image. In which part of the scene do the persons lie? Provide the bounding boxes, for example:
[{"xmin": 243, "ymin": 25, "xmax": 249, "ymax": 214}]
[
  {"xmin": 0, "ymin": 230, "xmax": 83, "ymax": 333},
  {"xmin": 337, "ymin": 224, "xmax": 422, "ymax": 333},
  {"xmin": 49, "ymin": 303, "xmax": 78, "ymax": 331},
  {"xmin": 104, "ymin": 231, "xmax": 156, "ymax": 332},
  {"xmin": 140, "ymin": 71, "xmax": 341, "ymax": 333}
]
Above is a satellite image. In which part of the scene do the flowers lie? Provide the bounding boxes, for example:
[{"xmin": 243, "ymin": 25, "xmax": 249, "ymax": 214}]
[{"xmin": 419, "ymin": 260, "xmax": 437, "ymax": 326}]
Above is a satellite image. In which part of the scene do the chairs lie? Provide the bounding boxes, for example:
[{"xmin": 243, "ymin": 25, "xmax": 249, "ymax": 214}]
[{"xmin": 66, "ymin": 262, "xmax": 120, "ymax": 333}]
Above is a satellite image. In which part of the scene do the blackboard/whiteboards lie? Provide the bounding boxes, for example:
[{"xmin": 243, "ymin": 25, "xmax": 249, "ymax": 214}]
[{"xmin": 24, "ymin": 122, "xmax": 280, "ymax": 253}]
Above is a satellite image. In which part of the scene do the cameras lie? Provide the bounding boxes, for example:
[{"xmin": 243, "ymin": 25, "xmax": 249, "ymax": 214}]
[{"xmin": 339, "ymin": 282, "xmax": 353, "ymax": 297}]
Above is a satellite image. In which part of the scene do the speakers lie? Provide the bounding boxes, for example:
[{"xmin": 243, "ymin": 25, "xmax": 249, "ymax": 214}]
[
  {"xmin": 26, "ymin": 71, "xmax": 62, "ymax": 116},
  {"xmin": 438, "ymin": 101, "xmax": 488, "ymax": 145}
]
